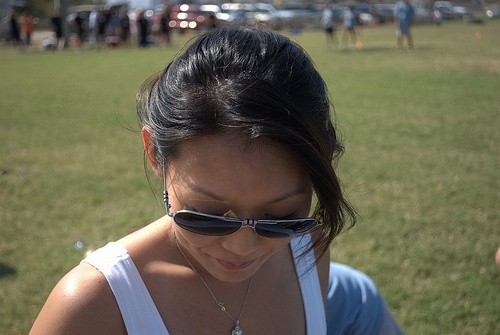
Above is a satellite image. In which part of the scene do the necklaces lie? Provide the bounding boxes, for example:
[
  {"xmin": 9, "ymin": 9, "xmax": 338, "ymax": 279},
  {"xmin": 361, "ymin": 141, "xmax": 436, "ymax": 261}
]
[{"xmin": 175, "ymin": 232, "xmax": 253, "ymax": 335}]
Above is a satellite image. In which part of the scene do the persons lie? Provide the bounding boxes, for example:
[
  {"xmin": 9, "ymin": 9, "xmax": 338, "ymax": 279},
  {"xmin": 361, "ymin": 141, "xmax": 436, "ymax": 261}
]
[
  {"xmin": 394, "ymin": 0, "xmax": 415, "ymax": 53},
  {"xmin": 28, "ymin": 27, "xmax": 403, "ymax": 335},
  {"xmin": 9, "ymin": 3, "xmax": 173, "ymax": 55},
  {"xmin": 320, "ymin": 1, "xmax": 360, "ymax": 48}
]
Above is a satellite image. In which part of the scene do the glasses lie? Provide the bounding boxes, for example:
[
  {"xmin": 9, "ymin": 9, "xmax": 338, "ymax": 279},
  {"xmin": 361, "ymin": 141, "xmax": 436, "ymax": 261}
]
[{"xmin": 162, "ymin": 151, "xmax": 325, "ymax": 239}]
